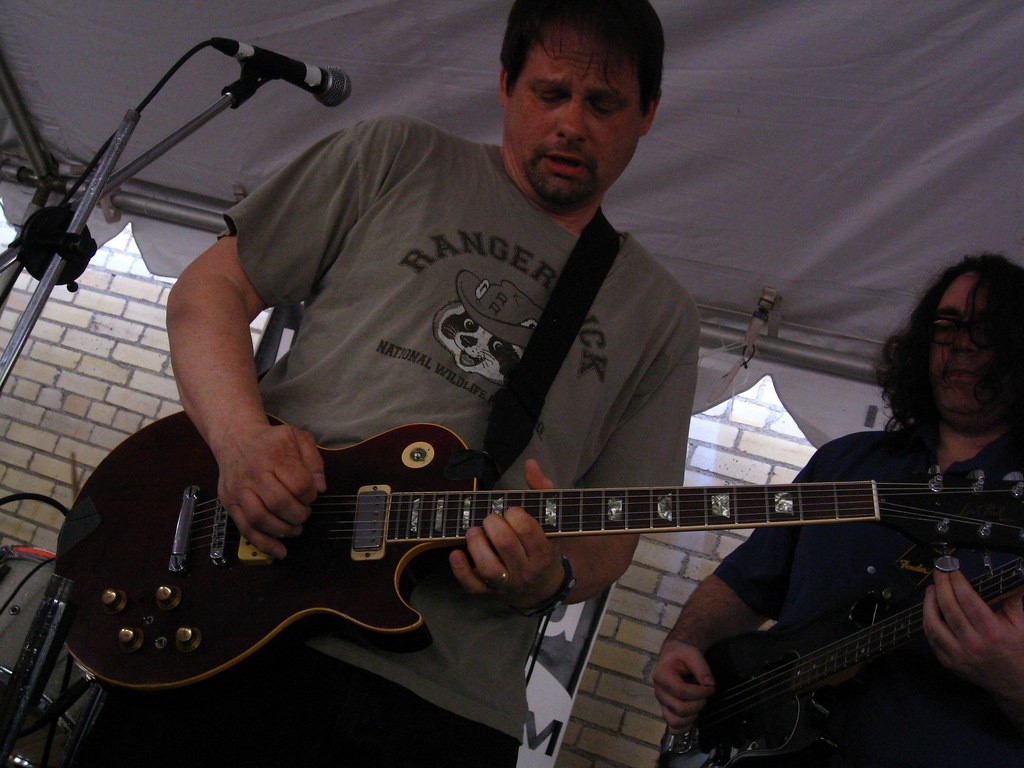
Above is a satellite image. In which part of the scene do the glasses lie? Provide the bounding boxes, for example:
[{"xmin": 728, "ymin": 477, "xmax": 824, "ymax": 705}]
[{"xmin": 926, "ymin": 316, "xmax": 996, "ymax": 348}]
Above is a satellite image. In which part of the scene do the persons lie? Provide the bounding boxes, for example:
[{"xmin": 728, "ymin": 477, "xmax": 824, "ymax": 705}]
[
  {"xmin": 66, "ymin": 0, "xmax": 700, "ymax": 768},
  {"xmin": 652, "ymin": 252, "xmax": 1024, "ymax": 768}
]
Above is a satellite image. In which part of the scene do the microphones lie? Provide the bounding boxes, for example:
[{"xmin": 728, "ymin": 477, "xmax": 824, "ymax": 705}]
[{"xmin": 211, "ymin": 36, "xmax": 353, "ymax": 106}]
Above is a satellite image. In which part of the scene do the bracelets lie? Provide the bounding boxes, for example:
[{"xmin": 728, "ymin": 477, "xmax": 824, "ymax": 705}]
[{"xmin": 508, "ymin": 554, "xmax": 577, "ymax": 618}]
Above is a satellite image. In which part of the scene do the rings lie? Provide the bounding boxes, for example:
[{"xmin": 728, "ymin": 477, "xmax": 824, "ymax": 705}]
[{"xmin": 500, "ymin": 570, "xmax": 510, "ymax": 584}]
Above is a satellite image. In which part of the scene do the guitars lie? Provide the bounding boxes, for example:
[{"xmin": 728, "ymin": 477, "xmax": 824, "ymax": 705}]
[
  {"xmin": 651, "ymin": 546, "xmax": 1024, "ymax": 768},
  {"xmin": 46, "ymin": 409, "xmax": 1024, "ymax": 697}
]
